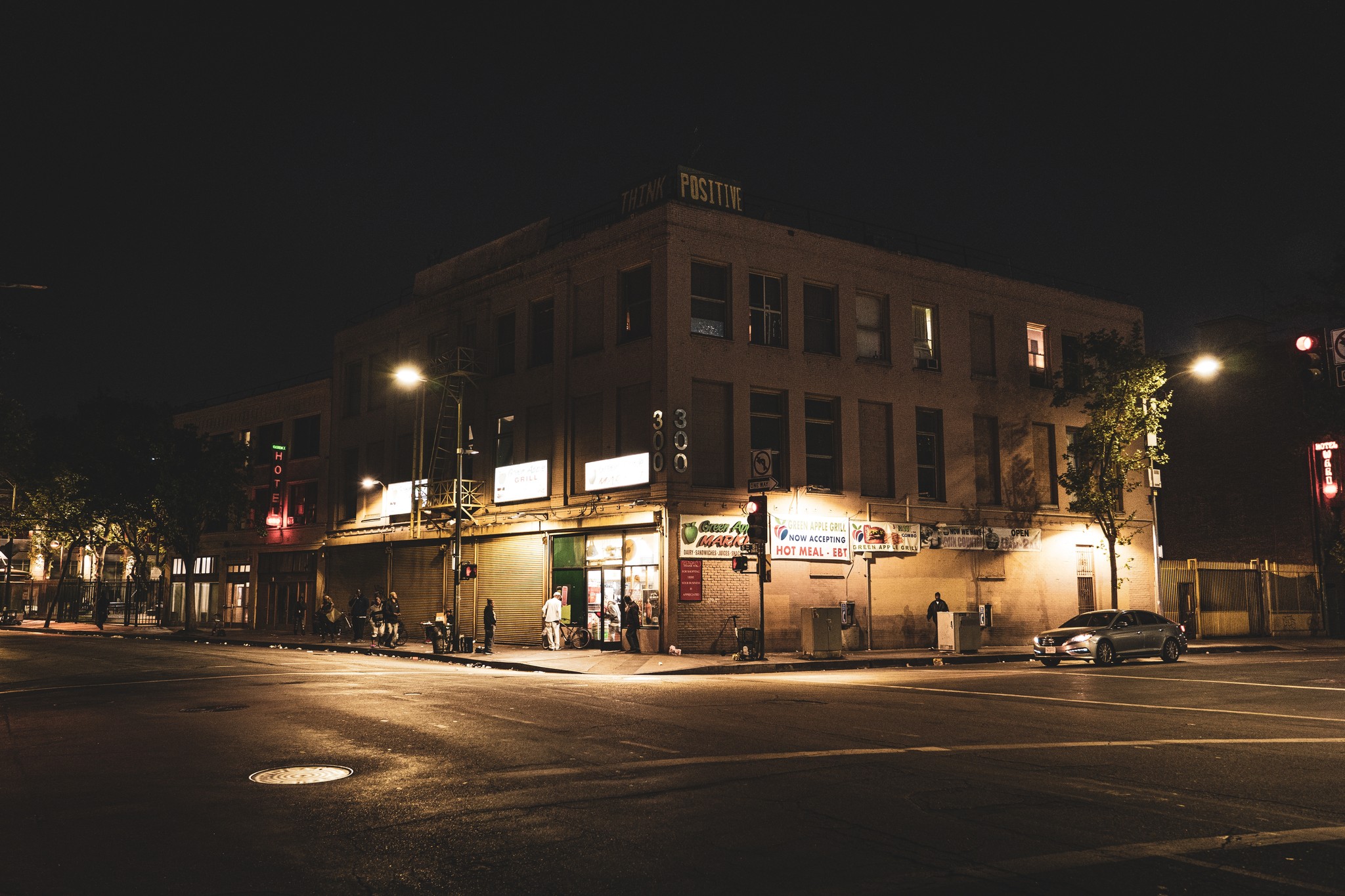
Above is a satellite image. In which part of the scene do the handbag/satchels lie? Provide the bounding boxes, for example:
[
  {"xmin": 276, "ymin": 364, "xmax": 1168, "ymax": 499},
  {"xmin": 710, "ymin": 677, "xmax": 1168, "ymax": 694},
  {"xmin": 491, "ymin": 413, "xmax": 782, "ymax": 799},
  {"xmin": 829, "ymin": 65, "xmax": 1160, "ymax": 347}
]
[
  {"xmin": 375, "ymin": 622, "xmax": 382, "ymax": 627},
  {"xmin": 326, "ymin": 608, "xmax": 342, "ymax": 623}
]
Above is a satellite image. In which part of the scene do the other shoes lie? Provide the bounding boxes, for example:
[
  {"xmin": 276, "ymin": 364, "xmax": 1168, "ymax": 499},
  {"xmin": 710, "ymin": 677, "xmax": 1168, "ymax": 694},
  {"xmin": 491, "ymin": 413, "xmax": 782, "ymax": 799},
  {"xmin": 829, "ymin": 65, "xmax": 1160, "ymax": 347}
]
[
  {"xmin": 332, "ymin": 639, "xmax": 335, "ymax": 643},
  {"xmin": 547, "ymin": 648, "xmax": 555, "ymax": 651},
  {"xmin": 426, "ymin": 639, "xmax": 432, "ymax": 643},
  {"xmin": 390, "ymin": 645, "xmax": 398, "ymax": 649},
  {"xmin": 321, "ymin": 640, "xmax": 325, "ymax": 643},
  {"xmin": 624, "ymin": 649, "xmax": 641, "ymax": 653},
  {"xmin": 928, "ymin": 647, "xmax": 936, "ymax": 650},
  {"xmin": 370, "ymin": 645, "xmax": 375, "ymax": 648},
  {"xmin": 555, "ymin": 647, "xmax": 561, "ymax": 650},
  {"xmin": 483, "ymin": 650, "xmax": 495, "ymax": 654}
]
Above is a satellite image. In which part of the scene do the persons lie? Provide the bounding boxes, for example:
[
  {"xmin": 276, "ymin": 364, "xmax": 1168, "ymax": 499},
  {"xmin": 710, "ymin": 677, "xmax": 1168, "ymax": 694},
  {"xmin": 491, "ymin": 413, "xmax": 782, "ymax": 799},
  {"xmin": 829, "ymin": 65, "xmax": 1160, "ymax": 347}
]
[
  {"xmin": 425, "ymin": 609, "xmax": 453, "ymax": 643},
  {"xmin": 368, "ymin": 592, "xmax": 385, "ymax": 647},
  {"xmin": 542, "ymin": 592, "xmax": 562, "ymax": 651},
  {"xmin": 312, "ymin": 596, "xmax": 342, "ymax": 642},
  {"xmin": 1103, "ymin": 613, "xmax": 1117, "ymax": 625},
  {"xmin": 623, "ymin": 595, "xmax": 641, "ymax": 654},
  {"xmin": 380, "ymin": 591, "xmax": 400, "ymax": 649},
  {"xmin": 483, "ymin": 598, "xmax": 497, "ymax": 655},
  {"xmin": 348, "ymin": 589, "xmax": 370, "ymax": 642},
  {"xmin": 604, "ymin": 597, "xmax": 641, "ymax": 641},
  {"xmin": 291, "ymin": 596, "xmax": 307, "ymax": 637},
  {"xmin": 644, "ymin": 597, "xmax": 652, "ymax": 625},
  {"xmin": 95, "ymin": 590, "xmax": 110, "ymax": 630},
  {"xmin": 926, "ymin": 591, "xmax": 949, "ymax": 650}
]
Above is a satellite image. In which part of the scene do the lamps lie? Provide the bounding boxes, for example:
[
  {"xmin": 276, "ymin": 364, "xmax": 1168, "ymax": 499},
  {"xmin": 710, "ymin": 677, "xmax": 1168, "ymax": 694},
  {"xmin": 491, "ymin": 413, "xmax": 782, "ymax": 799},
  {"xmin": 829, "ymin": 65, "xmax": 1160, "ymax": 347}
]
[
  {"xmin": 629, "ymin": 499, "xmax": 665, "ymax": 506},
  {"xmin": 935, "ymin": 523, "xmax": 946, "ymax": 528},
  {"xmin": 446, "ymin": 518, "xmax": 478, "ymax": 525},
  {"xmin": 373, "ymin": 480, "xmax": 387, "ymax": 490},
  {"xmin": 508, "ymin": 512, "xmax": 548, "ymax": 520}
]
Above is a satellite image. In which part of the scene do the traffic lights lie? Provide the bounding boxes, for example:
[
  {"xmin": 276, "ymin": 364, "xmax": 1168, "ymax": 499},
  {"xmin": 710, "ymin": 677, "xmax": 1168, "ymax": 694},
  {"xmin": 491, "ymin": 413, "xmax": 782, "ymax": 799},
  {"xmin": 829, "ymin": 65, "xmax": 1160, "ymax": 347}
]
[
  {"xmin": 746, "ymin": 495, "xmax": 768, "ymax": 544},
  {"xmin": 461, "ymin": 563, "xmax": 477, "ymax": 579},
  {"xmin": 732, "ymin": 554, "xmax": 748, "ymax": 573}
]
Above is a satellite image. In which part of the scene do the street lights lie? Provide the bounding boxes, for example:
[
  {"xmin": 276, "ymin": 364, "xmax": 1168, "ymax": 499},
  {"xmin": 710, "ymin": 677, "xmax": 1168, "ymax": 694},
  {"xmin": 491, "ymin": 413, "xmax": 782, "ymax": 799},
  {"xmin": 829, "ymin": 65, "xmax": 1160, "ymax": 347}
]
[
  {"xmin": 395, "ymin": 370, "xmax": 461, "ymax": 655},
  {"xmin": 50, "ymin": 540, "xmax": 64, "ymax": 580}
]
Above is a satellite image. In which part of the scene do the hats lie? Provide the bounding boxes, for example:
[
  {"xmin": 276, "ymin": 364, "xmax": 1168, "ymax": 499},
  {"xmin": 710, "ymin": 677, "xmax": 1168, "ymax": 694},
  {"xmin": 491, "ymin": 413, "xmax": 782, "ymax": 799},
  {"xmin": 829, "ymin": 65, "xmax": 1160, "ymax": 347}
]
[{"xmin": 553, "ymin": 592, "xmax": 563, "ymax": 598}]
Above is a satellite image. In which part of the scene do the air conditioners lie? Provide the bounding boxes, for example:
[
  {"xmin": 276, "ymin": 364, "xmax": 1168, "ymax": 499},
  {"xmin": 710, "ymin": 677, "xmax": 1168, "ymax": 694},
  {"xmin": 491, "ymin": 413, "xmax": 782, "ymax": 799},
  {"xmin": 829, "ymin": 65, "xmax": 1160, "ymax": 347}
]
[{"xmin": 924, "ymin": 358, "xmax": 938, "ymax": 369}]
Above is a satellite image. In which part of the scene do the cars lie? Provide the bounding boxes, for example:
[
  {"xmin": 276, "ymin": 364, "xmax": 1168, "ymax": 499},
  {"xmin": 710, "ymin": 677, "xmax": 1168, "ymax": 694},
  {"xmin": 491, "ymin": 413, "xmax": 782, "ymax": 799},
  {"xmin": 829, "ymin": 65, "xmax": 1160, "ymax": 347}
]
[{"xmin": 1033, "ymin": 608, "xmax": 1188, "ymax": 668}]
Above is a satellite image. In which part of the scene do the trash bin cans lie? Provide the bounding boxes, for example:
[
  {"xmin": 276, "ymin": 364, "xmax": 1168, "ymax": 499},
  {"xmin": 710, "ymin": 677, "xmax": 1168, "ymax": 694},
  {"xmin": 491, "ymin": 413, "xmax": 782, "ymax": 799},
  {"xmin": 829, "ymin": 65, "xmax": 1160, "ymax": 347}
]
[
  {"xmin": 428, "ymin": 624, "xmax": 454, "ymax": 654},
  {"xmin": 738, "ymin": 627, "xmax": 760, "ymax": 660},
  {"xmin": 4, "ymin": 609, "xmax": 17, "ymax": 625}
]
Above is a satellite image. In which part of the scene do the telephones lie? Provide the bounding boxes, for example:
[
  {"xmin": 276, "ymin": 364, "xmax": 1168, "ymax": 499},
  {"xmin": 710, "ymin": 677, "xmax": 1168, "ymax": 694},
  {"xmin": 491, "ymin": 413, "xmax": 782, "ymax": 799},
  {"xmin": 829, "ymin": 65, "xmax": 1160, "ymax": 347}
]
[
  {"xmin": 985, "ymin": 612, "xmax": 988, "ymax": 618},
  {"xmin": 847, "ymin": 609, "xmax": 851, "ymax": 616}
]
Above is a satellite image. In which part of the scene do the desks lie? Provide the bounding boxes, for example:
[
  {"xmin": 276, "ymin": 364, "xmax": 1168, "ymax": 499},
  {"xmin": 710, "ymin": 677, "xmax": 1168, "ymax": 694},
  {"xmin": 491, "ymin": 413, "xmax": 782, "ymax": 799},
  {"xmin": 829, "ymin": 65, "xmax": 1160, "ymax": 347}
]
[{"xmin": 415, "ymin": 623, "xmax": 433, "ymax": 643}]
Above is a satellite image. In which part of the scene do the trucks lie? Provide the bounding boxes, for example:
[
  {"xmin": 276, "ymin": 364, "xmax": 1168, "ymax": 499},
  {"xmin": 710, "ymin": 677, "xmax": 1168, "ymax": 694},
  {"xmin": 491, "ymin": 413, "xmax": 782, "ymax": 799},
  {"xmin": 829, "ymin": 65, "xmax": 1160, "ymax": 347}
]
[{"xmin": 0, "ymin": 613, "xmax": 24, "ymax": 622}]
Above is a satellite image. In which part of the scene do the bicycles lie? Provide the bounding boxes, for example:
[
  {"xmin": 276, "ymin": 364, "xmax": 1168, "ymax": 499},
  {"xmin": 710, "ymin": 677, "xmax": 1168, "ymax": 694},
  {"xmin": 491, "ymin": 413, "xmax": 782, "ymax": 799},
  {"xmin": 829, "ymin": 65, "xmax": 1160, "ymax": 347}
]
[
  {"xmin": 542, "ymin": 617, "xmax": 592, "ymax": 650},
  {"xmin": 389, "ymin": 618, "xmax": 408, "ymax": 646},
  {"xmin": 211, "ymin": 618, "xmax": 226, "ymax": 636}
]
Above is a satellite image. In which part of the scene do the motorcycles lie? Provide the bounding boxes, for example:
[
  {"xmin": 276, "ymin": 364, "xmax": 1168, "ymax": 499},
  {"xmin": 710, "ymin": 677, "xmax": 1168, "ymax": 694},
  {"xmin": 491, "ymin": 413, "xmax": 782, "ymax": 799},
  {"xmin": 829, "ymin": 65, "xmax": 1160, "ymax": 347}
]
[{"xmin": 0, "ymin": 609, "xmax": 22, "ymax": 626}]
